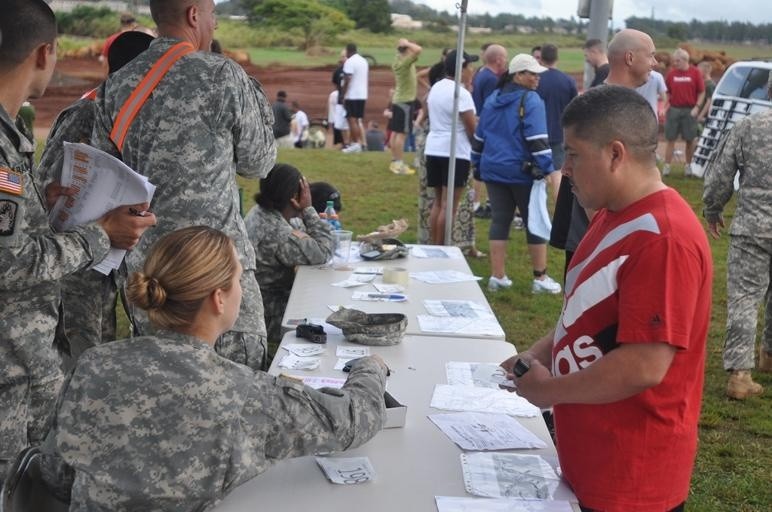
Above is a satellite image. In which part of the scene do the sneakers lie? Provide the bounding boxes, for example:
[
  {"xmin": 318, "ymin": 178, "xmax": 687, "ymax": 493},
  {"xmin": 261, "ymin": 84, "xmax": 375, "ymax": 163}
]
[
  {"xmin": 662, "ymin": 163, "xmax": 671, "ymax": 175},
  {"xmin": 393, "ymin": 163, "xmax": 415, "ymax": 175},
  {"xmin": 513, "ymin": 213, "xmax": 524, "ymax": 230},
  {"xmin": 341, "ymin": 142, "xmax": 361, "ymax": 154},
  {"xmin": 683, "ymin": 163, "xmax": 691, "ymax": 177},
  {"xmin": 531, "ymin": 277, "xmax": 562, "ymax": 295},
  {"xmin": 487, "ymin": 276, "xmax": 513, "ymax": 292}
]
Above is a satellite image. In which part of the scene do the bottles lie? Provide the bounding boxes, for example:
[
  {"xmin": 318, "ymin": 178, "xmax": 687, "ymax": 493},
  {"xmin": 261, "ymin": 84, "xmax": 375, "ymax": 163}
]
[{"xmin": 317, "ymin": 200, "xmax": 342, "ymax": 231}]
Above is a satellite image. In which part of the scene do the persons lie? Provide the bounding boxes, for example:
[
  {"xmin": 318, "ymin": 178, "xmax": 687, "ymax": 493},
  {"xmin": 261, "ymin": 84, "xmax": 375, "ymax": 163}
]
[
  {"xmin": 701, "ymin": 80, "xmax": 772, "ymax": 402},
  {"xmin": 98, "ymin": 12, "xmax": 158, "ymax": 78},
  {"xmin": 0, "ymin": 0, "xmax": 160, "ymax": 494},
  {"xmin": 549, "ymin": 29, "xmax": 658, "ymax": 281},
  {"xmin": 418, "ymin": 44, "xmax": 578, "ymax": 294},
  {"xmin": 210, "ymin": 36, "xmax": 418, "ymax": 181},
  {"xmin": 500, "ymin": 83, "xmax": 714, "ymax": 511},
  {"xmin": 34, "ymin": 30, "xmax": 155, "ymax": 356},
  {"xmin": 37, "ymin": 222, "xmax": 396, "ymax": 511},
  {"xmin": 90, "ymin": 2, "xmax": 280, "ymax": 373},
  {"xmin": 639, "ymin": 49, "xmax": 715, "ymax": 176},
  {"xmin": 240, "ymin": 162, "xmax": 338, "ymax": 343}
]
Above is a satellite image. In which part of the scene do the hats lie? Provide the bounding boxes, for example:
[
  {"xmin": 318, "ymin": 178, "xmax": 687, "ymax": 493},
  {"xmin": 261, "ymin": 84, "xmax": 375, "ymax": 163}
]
[
  {"xmin": 508, "ymin": 53, "xmax": 548, "ymax": 75},
  {"xmin": 444, "ymin": 50, "xmax": 479, "ymax": 74},
  {"xmin": 359, "ymin": 238, "xmax": 408, "ymax": 260},
  {"xmin": 325, "ymin": 307, "xmax": 408, "ymax": 346}
]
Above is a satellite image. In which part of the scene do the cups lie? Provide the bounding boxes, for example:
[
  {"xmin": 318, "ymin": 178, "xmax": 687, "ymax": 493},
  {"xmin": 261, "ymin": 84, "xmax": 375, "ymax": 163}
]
[{"xmin": 329, "ymin": 230, "xmax": 353, "ymax": 270}]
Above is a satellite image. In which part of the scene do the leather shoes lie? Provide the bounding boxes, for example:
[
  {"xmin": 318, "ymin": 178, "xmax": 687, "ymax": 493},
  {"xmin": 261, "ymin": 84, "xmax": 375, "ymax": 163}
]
[
  {"xmin": 726, "ymin": 375, "xmax": 764, "ymax": 400},
  {"xmin": 759, "ymin": 350, "xmax": 772, "ymax": 370}
]
